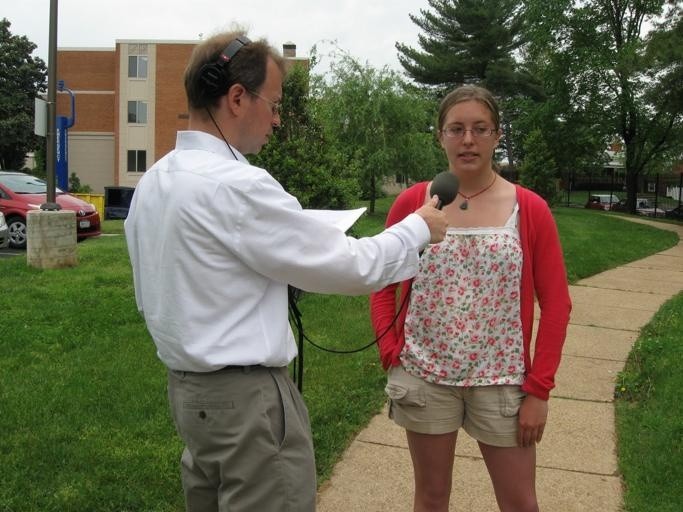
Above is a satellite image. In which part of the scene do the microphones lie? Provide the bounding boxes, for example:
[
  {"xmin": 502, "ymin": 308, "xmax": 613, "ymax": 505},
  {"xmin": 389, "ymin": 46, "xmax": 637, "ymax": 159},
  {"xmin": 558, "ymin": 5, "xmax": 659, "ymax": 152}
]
[{"xmin": 419, "ymin": 171, "xmax": 459, "ymax": 256}]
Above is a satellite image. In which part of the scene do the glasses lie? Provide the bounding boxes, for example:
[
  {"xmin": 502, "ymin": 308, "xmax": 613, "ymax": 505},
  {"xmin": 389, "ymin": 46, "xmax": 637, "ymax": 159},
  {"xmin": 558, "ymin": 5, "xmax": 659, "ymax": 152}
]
[
  {"xmin": 440, "ymin": 126, "xmax": 497, "ymax": 138},
  {"xmin": 248, "ymin": 89, "xmax": 283, "ymax": 112}
]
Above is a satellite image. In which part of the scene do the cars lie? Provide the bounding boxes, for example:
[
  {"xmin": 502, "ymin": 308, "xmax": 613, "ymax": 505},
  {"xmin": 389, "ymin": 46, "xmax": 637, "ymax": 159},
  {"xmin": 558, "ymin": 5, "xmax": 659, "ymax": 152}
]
[
  {"xmin": 0, "ymin": 170, "xmax": 101, "ymax": 250},
  {"xmin": 585, "ymin": 194, "xmax": 620, "ymax": 211},
  {"xmin": 611, "ymin": 198, "xmax": 666, "ymax": 218},
  {"xmin": 666, "ymin": 205, "xmax": 683, "ymax": 220}
]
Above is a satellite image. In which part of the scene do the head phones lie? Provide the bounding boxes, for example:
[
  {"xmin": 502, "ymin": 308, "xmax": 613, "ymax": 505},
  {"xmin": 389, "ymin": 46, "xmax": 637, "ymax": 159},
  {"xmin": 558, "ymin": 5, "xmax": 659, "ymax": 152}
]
[{"xmin": 195, "ymin": 35, "xmax": 251, "ymax": 97}]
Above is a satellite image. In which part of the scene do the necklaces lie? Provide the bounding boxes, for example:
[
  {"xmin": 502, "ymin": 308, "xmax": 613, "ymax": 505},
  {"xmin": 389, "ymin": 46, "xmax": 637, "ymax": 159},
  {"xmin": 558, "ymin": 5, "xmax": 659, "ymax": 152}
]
[{"xmin": 455, "ymin": 172, "xmax": 497, "ymax": 212}]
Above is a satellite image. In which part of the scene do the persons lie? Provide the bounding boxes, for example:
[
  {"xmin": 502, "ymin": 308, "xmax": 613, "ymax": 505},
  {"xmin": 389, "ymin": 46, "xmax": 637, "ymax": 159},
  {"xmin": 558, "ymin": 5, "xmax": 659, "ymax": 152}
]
[
  {"xmin": 121, "ymin": 29, "xmax": 451, "ymax": 512},
  {"xmin": 365, "ymin": 82, "xmax": 572, "ymax": 512}
]
